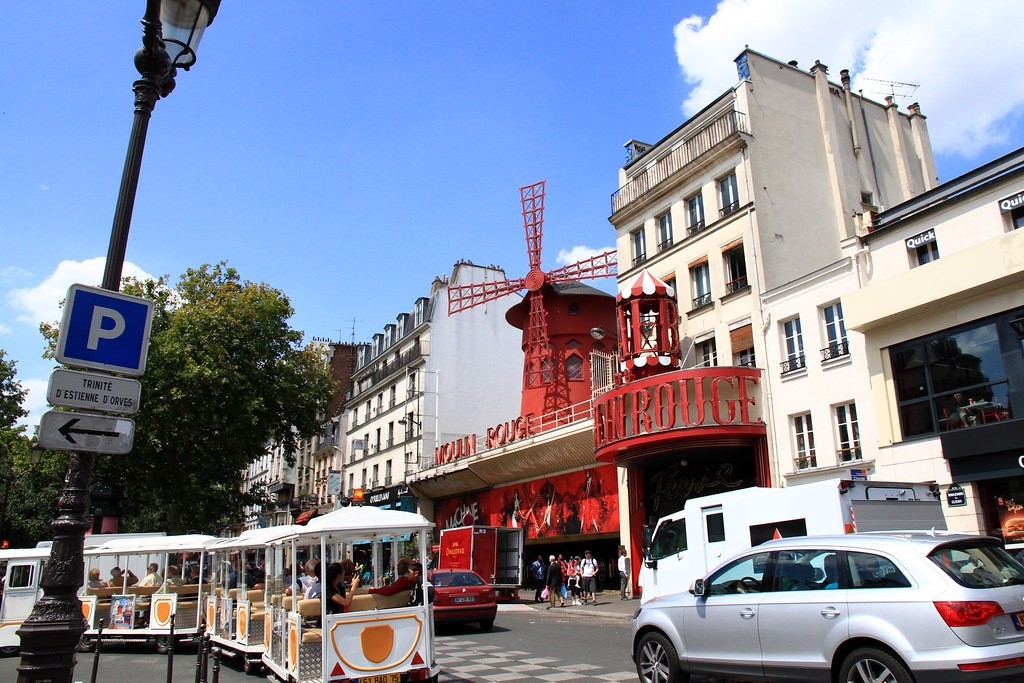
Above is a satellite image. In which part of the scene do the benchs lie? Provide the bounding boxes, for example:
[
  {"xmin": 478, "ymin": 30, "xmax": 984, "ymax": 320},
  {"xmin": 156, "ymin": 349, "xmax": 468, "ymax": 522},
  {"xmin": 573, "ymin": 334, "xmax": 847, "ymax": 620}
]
[
  {"xmin": 282, "ymin": 587, "xmax": 373, "ymax": 613},
  {"xmin": 169, "ymin": 584, "xmax": 211, "ymax": 610},
  {"xmin": 215, "ymin": 587, "xmax": 222, "ymax": 612},
  {"xmin": 229, "ymin": 587, "xmax": 252, "ymax": 618},
  {"xmin": 864, "ymin": 572, "xmax": 911, "ymax": 588},
  {"xmin": 87, "ymin": 586, "xmax": 123, "ymax": 611},
  {"xmin": 247, "ymin": 589, "xmax": 265, "ymax": 620},
  {"xmin": 126, "ymin": 586, "xmax": 161, "ymax": 610},
  {"xmin": 297, "ymin": 591, "xmax": 412, "ymax": 643}
]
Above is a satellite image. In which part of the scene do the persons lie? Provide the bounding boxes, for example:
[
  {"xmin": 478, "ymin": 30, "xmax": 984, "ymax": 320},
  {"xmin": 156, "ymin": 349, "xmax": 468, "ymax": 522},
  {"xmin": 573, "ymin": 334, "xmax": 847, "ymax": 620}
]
[
  {"xmin": 618, "ymin": 550, "xmax": 629, "ymax": 600},
  {"xmin": 530, "ymin": 555, "xmax": 546, "ymax": 603},
  {"xmin": 139, "ymin": 563, "xmax": 164, "ymax": 585},
  {"xmin": 369, "ymin": 556, "xmax": 435, "ymax": 608},
  {"xmin": 154, "ymin": 565, "xmax": 207, "ymax": 597},
  {"xmin": 736, "ymin": 553, "xmax": 793, "ymax": 593},
  {"xmin": 228, "ymin": 559, "xmax": 366, "ymax": 627},
  {"xmin": 109, "ymin": 567, "xmax": 138, "ymax": 586},
  {"xmin": 546, "ymin": 551, "xmax": 599, "ymax": 607},
  {"xmin": 948, "ymin": 393, "xmax": 976, "ymax": 428},
  {"xmin": 79, "ymin": 569, "xmax": 109, "ymax": 596}
]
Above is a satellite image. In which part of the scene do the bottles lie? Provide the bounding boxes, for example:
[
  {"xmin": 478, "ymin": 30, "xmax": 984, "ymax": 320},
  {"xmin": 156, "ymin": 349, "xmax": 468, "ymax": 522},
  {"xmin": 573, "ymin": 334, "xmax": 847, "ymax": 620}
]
[
  {"xmin": 124, "ymin": 606, "xmax": 131, "ymax": 624},
  {"xmin": 115, "ymin": 606, "xmax": 124, "ymax": 624}
]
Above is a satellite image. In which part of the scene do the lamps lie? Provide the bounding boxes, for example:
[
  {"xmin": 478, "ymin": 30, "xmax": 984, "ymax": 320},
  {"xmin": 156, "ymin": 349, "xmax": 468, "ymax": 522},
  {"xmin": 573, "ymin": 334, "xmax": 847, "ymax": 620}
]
[
  {"xmin": 590, "ymin": 325, "xmax": 617, "ymax": 341},
  {"xmin": 298, "ymin": 464, "xmax": 314, "ymax": 471},
  {"xmin": 398, "ymin": 417, "xmax": 422, "ymax": 429}
]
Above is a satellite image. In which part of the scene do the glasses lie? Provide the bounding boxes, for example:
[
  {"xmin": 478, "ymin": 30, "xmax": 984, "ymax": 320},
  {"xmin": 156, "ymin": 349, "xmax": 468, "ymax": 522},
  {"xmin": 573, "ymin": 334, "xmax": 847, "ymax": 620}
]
[{"xmin": 575, "ymin": 558, "xmax": 579, "ymax": 560}]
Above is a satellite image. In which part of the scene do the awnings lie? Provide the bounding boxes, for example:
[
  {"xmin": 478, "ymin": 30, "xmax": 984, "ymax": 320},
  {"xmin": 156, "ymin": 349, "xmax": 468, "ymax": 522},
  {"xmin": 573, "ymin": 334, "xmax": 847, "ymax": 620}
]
[{"xmin": 294, "ymin": 509, "xmax": 316, "ymax": 523}]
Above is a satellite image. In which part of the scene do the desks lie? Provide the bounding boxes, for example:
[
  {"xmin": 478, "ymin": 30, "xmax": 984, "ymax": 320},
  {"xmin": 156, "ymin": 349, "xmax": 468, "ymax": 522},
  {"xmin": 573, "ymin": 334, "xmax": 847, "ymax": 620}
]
[{"xmin": 959, "ymin": 402, "xmax": 991, "ymax": 426}]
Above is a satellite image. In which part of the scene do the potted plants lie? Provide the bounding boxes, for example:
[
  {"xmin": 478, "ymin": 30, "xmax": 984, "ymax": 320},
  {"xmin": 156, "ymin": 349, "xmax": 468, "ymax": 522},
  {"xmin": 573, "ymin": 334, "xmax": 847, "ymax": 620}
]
[{"xmin": 313, "ymin": 445, "xmax": 335, "ymax": 461}]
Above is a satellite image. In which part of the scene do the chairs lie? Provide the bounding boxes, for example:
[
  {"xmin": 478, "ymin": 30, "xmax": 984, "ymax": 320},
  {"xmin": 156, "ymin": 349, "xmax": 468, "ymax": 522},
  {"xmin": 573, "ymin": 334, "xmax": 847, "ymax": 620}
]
[
  {"xmin": 942, "ymin": 395, "xmax": 1010, "ymax": 431},
  {"xmin": 450, "ymin": 575, "xmax": 466, "ymax": 586},
  {"xmin": 820, "ymin": 554, "xmax": 837, "ymax": 590},
  {"xmin": 780, "ymin": 560, "xmax": 813, "ymax": 591}
]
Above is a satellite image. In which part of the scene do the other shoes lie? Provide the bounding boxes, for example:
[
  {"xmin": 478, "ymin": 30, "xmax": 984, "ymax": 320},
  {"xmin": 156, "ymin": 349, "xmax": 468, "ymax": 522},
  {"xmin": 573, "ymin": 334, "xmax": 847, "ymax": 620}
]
[
  {"xmin": 541, "ymin": 598, "xmax": 544, "ymax": 602},
  {"xmin": 548, "ymin": 605, "xmax": 555, "ymax": 608},
  {"xmin": 558, "ymin": 601, "xmax": 564, "ymax": 607},
  {"xmin": 567, "ymin": 583, "xmax": 570, "ymax": 587},
  {"xmin": 571, "ymin": 601, "xmax": 576, "ymax": 605},
  {"xmin": 584, "ymin": 599, "xmax": 587, "ymax": 605},
  {"xmin": 535, "ymin": 600, "xmax": 539, "ymax": 603},
  {"xmin": 575, "ymin": 585, "xmax": 581, "ymax": 589},
  {"xmin": 592, "ymin": 600, "xmax": 596, "ymax": 606},
  {"xmin": 575, "ymin": 601, "xmax": 581, "ymax": 606}
]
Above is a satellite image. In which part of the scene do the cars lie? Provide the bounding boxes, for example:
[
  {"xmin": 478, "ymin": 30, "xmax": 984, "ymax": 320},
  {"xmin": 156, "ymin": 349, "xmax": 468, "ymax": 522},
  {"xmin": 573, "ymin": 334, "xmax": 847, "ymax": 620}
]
[
  {"xmin": 630, "ymin": 526, "xmax": 1024, "ymax": 683},
  {"xmin": 426, "ymin": 569, "xmax": 498, "ymax": 634}
]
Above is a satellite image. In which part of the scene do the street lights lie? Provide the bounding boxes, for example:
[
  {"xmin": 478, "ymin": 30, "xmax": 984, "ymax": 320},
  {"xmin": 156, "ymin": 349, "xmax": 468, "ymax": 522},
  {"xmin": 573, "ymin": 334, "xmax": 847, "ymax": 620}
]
[{"xmin": 14, "ymin": 0, "xmax": 221, "ymax": 683}]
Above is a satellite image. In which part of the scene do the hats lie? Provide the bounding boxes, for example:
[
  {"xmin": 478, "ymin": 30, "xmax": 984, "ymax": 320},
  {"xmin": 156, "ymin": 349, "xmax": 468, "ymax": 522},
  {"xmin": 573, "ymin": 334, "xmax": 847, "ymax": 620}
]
[
  {"xmin": 575, "ymin": 556, "xmax": 580, "ymax": 560},
  {"xmin": 584, "ymin": 550, "xmax": 591, "ymax": 553}
]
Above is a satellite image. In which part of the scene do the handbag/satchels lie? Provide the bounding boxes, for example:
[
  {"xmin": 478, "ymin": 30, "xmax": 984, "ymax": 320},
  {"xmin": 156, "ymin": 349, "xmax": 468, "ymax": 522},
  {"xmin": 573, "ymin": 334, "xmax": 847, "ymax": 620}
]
[
  {"xmin": 560, "ymin": 583, "xmax": 568, "ymax": 599},
  {"xmin": 540, "ymin": 588, "xmax": 549, "ymax": 599}
]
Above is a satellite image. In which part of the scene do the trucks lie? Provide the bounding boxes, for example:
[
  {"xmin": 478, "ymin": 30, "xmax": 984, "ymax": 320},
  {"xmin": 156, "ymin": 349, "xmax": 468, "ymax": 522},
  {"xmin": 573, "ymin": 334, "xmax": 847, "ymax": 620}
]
[
  {"xmin": 638, "ymin": 479, "xmax": 949, "ymax": 604},
  {"xmin": 427, "ymin": 525, "xmax": 524, "ymax": 602}
]
